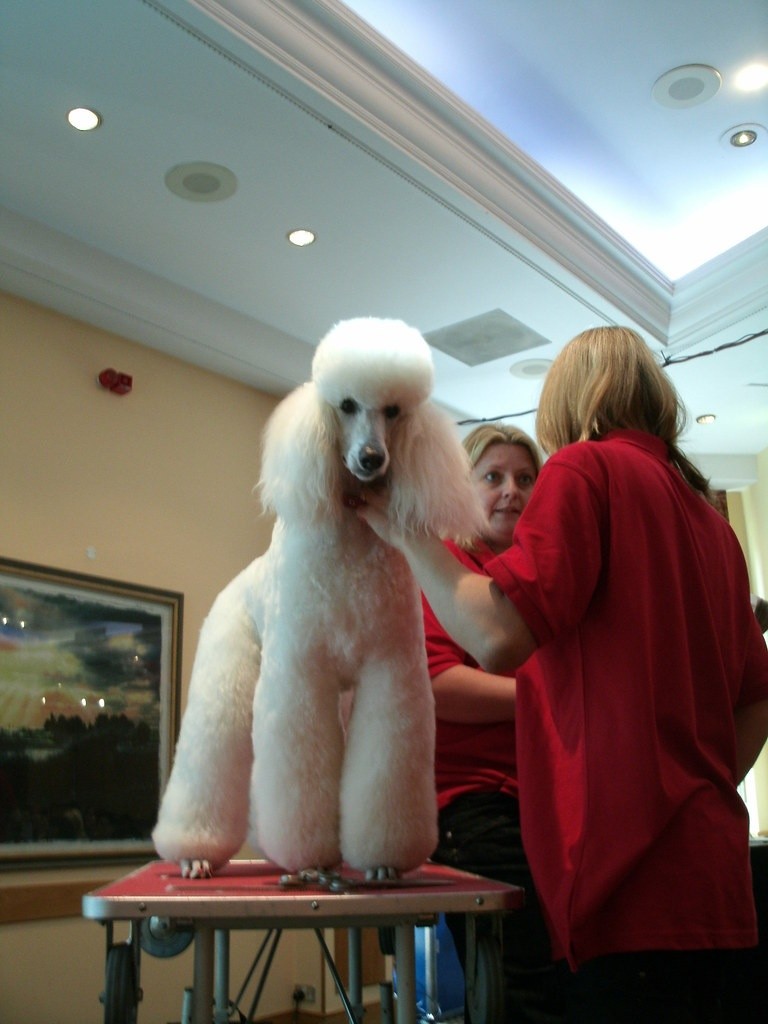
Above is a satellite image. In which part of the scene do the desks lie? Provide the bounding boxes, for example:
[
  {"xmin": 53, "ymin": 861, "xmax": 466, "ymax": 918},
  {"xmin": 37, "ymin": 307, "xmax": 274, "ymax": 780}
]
[{"xmin": 83, "ymin": 860, "xmax": 523, "ymax": 1024}]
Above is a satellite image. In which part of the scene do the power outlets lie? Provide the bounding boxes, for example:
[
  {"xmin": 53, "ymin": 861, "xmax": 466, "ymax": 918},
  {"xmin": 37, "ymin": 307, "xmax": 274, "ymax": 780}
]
[{"xmin": 294, "ymin": 984, "xmax": 316, "ymax": 1002}]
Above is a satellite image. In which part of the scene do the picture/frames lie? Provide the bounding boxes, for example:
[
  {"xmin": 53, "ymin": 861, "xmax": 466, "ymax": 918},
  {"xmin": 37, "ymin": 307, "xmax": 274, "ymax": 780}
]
[{"xmin": 0, "ymin": 555, "xmax": 185, "ymax": 863}]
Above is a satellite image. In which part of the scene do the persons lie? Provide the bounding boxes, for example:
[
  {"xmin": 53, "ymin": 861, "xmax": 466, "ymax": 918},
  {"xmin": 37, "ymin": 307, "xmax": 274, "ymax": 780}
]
[
  {"xmin": 418, "ymin": 422, "xmax": 550, "ymax": 1024},
  {"xmin": 352, "ymin": 325, "xmax": 768, "ymax": 1024}
]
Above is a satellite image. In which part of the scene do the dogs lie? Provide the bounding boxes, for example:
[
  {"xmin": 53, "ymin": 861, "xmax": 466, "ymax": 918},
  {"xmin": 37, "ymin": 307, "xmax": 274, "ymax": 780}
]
[{"xmin": 150, "ymin": 316, "xmax": 487, "ymax": 891}]
